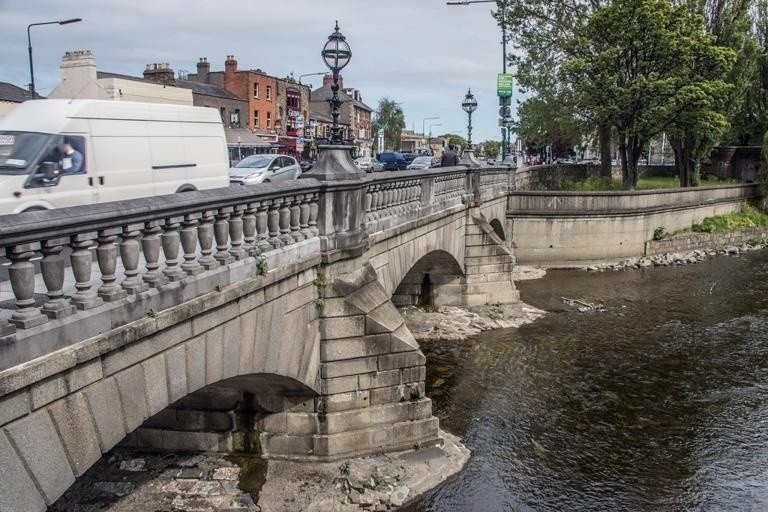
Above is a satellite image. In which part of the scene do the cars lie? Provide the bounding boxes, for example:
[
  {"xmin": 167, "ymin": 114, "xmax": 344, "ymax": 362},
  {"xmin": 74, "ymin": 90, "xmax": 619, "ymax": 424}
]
[
  {"xmin": 228, "ymin": 150, "xmax": 302, "ymax": 192},
  {"xmin": 512, "ymin": 153, "xmax": 622, "ymax": 167},
  {"xmin": 379, "ymin": 152, "xmax": 410, "ymax": 171},
  {"xmin": 354, "ymin": 156, "xmax": 384, "ymax": 173},
  {"xmin": 401, "ymin": 151, "xmax": 460, "ymax": 171}
]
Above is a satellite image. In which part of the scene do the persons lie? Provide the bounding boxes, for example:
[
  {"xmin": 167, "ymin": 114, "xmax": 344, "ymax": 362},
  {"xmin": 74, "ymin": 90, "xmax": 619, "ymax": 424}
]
[
  {"xmin": 54, "ymin": 141, "xmax": 84, "ymax": 176},
  {"xmin": 441, "ymin": 143, "xmax": 459, "ymax": 167}
]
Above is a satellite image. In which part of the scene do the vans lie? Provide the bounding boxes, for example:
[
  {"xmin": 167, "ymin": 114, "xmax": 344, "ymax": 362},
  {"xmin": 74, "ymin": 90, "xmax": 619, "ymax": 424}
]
[{"xmin": 0, "ymin": 99, "xmax": 231, "ymax": 216}]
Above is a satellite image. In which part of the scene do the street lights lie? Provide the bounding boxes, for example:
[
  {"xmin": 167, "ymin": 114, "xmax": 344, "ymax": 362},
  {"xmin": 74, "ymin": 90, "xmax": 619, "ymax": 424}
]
[
  {"xmin": 444, "ymin": 0, "xmax": 510, "ymax": 169},
  {"xmin": 462, "ymin": 88, "xmax": 478, "ymax": 150},
  {"xmin": 320, "ymin": 16, "xmax": 352, "ymax": 145},
  {"xmin": 421, "ymin": 115, "xmax": 461, "ymax": 146},
  {"xmin": 295, "ymin": 71, "xmax": 332, "ymax": 161},
  {"xmin": 380, "ymin": 101, "xmax": 403, "ymax": 130},
  {"xmin": 27, "ymin": 18, "xmax": 83, "ymax": 99}
]
